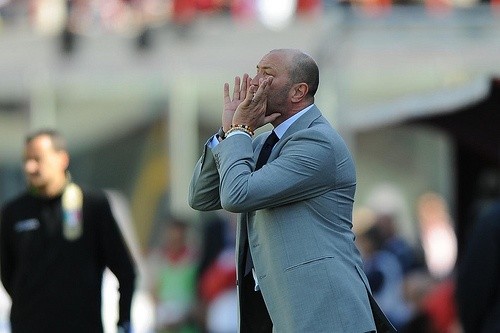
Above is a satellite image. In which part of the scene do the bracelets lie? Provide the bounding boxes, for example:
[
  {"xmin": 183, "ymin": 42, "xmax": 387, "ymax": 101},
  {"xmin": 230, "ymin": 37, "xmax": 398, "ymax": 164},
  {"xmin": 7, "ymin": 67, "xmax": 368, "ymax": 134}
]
[{"xmin": 224, "ymin": 123, "xmax": 256, "ymax": 137}]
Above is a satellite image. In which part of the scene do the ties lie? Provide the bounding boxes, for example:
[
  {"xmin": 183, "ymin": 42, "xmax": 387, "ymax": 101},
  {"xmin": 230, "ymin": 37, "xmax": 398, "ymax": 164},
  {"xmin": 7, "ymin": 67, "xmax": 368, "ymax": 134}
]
[{"xmin": 255, "ymin": 131, "xmax": 278, "ymax": 173}]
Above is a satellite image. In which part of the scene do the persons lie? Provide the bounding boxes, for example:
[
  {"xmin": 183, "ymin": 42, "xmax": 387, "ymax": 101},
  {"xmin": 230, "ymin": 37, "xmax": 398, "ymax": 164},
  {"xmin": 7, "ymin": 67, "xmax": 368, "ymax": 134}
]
[
  {"xmin": 188, "ymin": 48, "xmax": 395, "ymax": 333},
  {"xmin": 1, "ymin": 128, "xmax": 137, "ymax": 333}
]
